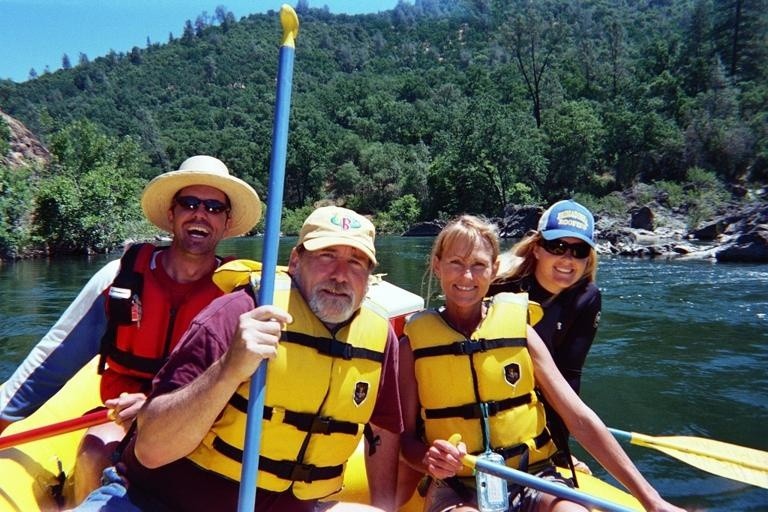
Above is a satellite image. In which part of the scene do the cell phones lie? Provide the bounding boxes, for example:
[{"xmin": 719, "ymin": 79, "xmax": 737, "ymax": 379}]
[{"xmin": 475, "ymin": 452, "xmax": 509, "ymax": 512}]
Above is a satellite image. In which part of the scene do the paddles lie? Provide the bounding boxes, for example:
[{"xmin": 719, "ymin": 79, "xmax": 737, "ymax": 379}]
[{"xmin": 570, "ymin": 427, "xmax": 768, "ymax": 490}]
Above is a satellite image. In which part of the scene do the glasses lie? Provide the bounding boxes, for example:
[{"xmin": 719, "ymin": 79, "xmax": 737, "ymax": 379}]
[
  {"xmin": 176, "ymin": 192, "xmax": 229, "ymax": 214},
  {"xmin": 539, "ymin": 241, "xmax": 592, "ymax": 260}
]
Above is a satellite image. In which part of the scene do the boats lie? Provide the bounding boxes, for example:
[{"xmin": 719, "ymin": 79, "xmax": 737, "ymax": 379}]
[{"xmin": 1, "ymin": 260, "xmax": 656, "ymax": 511}]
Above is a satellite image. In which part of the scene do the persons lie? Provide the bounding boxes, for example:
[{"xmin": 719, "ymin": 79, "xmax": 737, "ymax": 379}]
[
  {"xmin": 0, "ymin": 156, "xmax": 262, "ymax": 507},
  {"xmin": 396, "ymin": 212, "xmax": 689, "ymax": 511},
  {"xmin": 485, "ymin": 199, "xmax": 602, "ymax": 476},
  {"xmin": 70, "ymin": 205, "xmax": 404, "ymax": 511}
]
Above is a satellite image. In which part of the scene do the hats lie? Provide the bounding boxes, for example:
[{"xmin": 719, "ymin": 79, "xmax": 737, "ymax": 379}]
[
  {"xmin": 140, "ymin": 154, "xmax": 262, "ymax": 239},
  {"xmin": 538, "ymin": 198, "xmax": 597, "ymax": 251},
  {"xmin": 298, "ymin": 206, "xmax": 380, "ymax": 267}
]
